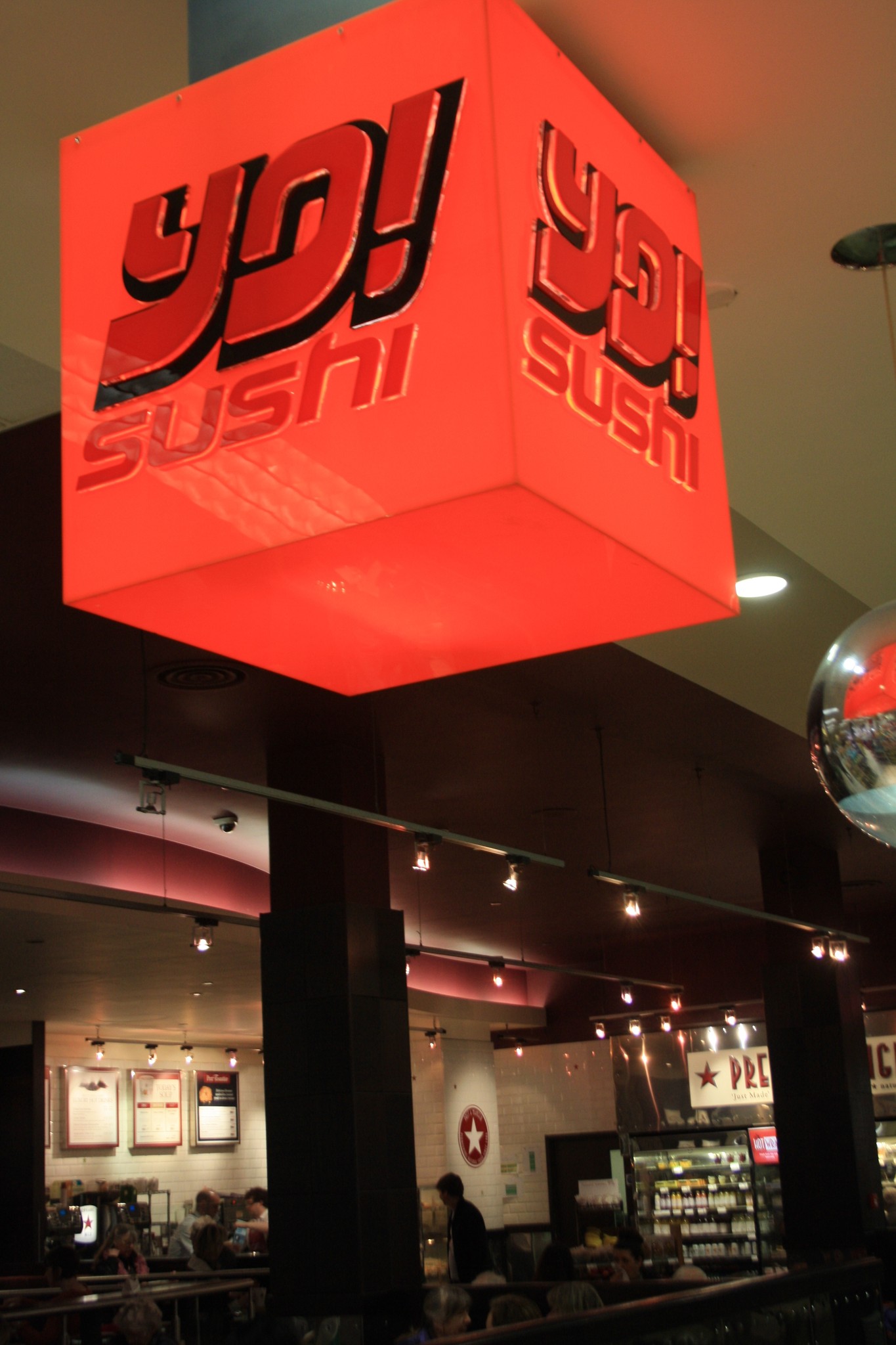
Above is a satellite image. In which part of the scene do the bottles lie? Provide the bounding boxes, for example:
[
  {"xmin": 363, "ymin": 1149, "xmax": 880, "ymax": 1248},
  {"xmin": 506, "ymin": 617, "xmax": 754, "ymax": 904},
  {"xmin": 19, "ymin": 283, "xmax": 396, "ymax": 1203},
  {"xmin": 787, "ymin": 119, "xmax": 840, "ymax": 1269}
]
[{"xmin": 650, "ymin": 1181, "xmax": 775, "ymax": 1259}]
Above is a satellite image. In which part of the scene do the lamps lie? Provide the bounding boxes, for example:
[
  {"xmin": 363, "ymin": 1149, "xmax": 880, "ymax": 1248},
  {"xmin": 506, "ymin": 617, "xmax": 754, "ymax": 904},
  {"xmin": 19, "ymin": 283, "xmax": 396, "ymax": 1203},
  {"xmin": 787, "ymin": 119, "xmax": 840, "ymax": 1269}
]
[
  {"xmin": 225, "ymin": 1048, "xmax": 237, "ymax": 1067},
  {"xmin": 502, "ymin": 855, "xmax": 528, "ymax": 890},
  {"xmin": 412, "ymin": 836, "xmax": 441, "ymax": 870},
  {"xmin": 619, "ymin": 982, "xmax": 635, "ymax": 1005},
  {"xmin": 145, "ymin": 1044, "xmax": 193, "ymax": 1064},
  {"xmin": 623, "ymin": 885, "xmax": 646, "ymax": 916},
  {"xmin": 594, "ymin": 1016, "xmax": 671, "ymax": 1039},
  {"xmin": 137, "ymin": 771, "xmax": 166, "ymax": 815},
  {"xmin": 721, "ymin": 1007, "xmax": 737, "ymax": 1026},
  {"xmin": 669, "ymin": 989, "xmax": 685, "ymax": 1009},
  {"xmin": 91, "ymin": 1041, "xmax": 105, "ymax": 1057},
  {"xmin": 809, "ymin": 931, "xmax": 850, "ymax": 961},
  {"xmin": 191, "ymin": 917, "xmax": 219, "ymax": 950}
]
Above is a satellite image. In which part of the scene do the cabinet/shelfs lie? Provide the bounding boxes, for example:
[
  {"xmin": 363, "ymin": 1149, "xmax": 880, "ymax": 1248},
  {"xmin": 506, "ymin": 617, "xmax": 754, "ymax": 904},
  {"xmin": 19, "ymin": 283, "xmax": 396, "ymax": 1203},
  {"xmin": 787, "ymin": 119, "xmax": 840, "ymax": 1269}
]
[{"xmin": 624, "ymin": 1138, "xmax": 784, "ymax": 1279}]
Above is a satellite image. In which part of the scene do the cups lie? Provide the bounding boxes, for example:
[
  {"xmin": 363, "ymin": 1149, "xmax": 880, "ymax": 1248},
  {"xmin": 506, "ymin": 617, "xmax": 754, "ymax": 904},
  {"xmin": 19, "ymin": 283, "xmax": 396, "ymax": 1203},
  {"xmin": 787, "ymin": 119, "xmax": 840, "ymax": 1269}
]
[
  {"xmin": 708, "ymin": 1176, "xmax": 717, "ymax": 1184},
  {"xmin": 657, "ymin": 1153, "xmax": 746, "ymax": 1171},
  {"xmin": 719, "ymin": 1176, "xmax": 726, "ymax": 1184},
  {"xmin": 728, "ymin": 1175, "xmax": 739, "ymax": 1183},
  {"xmin": 740, "ymin": 1173, "xmax": 751, "ymax": 1181}
]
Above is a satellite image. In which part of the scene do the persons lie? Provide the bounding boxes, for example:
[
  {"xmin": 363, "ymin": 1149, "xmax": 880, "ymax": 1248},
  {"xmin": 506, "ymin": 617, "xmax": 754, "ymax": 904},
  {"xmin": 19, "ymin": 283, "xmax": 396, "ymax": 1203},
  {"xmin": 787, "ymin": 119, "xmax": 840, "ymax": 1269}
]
[
  {"xmin": 0, "ymin": 1246, "xmax": 92, "ymax": 1345},
  {"xmin": 226, "ymin": 1281, "xmax": 309, "ymax": 1345},
  {"xmin": 106, "ymin": 1296, "xmax": 178, "ymax": 1345},
  {"xmin": 486, "ymin": 1294, "xmax": 541, "ymax": 1328},
  {"xmin": 436, "ymin": 1172, "xmax": 493, "ymax": 1283},
  {"xmin": 187, "ymin": 1215, "xmax": 249, "ymax": 1322},
  {"xmin": 398, "ymin": 1285, "xmax": 472, "ymax": 1345},
  {"xmin": 93, "ymin": 1223, "xmax": 149, "ymax": 1275},
  {"xmin": 601, "ymin": 1229, "xmax": 661, "ymax": 1281},
  {"xmin": 546, "ymin": 1282, "xmax": 604, "ymax": 1317},
  {"xmin": 167, "ymin": 1189, "xmax": 219, "ymax": 1256},
  {"xmin": 531, "ymin": 1242, "xmax": 575, "ymax": 1281},
  {"xmin": 232, "ymin": 1187, "xmax": 269, "ymax": 1238}
]
